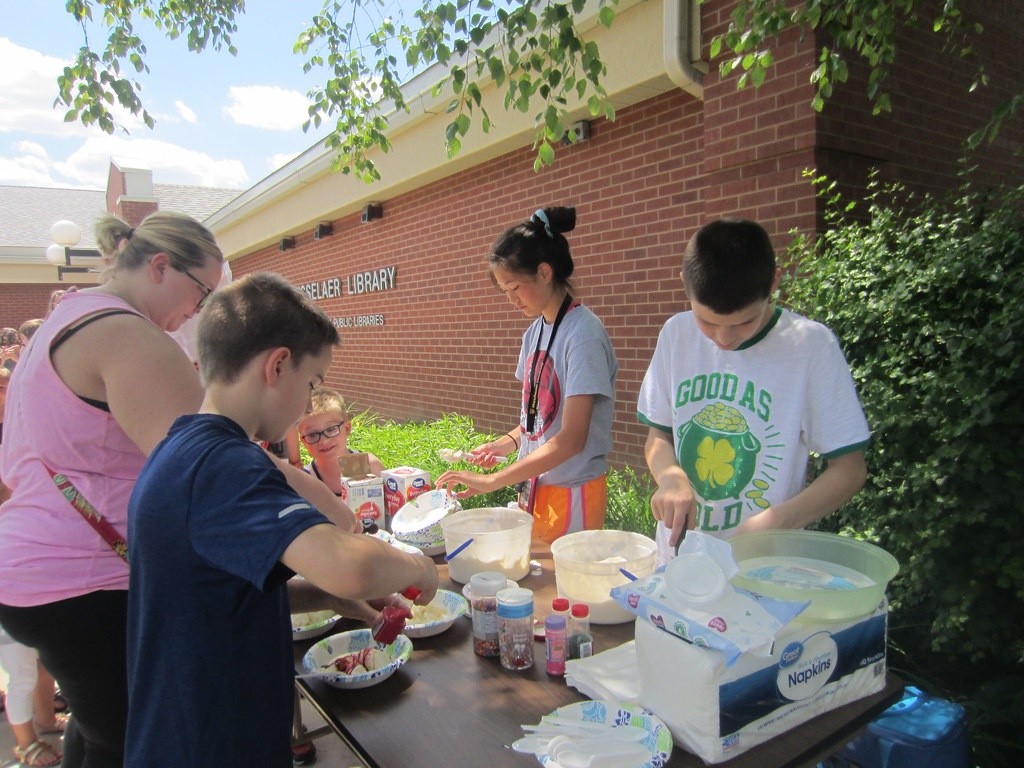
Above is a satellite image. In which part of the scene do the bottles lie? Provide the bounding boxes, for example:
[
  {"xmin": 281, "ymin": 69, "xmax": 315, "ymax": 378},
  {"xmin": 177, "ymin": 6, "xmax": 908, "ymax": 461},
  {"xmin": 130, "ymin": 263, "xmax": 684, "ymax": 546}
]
[
  {"xmin": 372, "ymin": 586, "xmax": 421, "ymax": 644},
  {"xmin": 544, "ymin": 598, "xmax": 594, "ymax": 676},
  {"xmin": 495, "ymin": 587, "xmax": 537, "ymax": 672},
  {"xmin": 462, "ymin": 579, "xmax": 520, "ymax": 657}
]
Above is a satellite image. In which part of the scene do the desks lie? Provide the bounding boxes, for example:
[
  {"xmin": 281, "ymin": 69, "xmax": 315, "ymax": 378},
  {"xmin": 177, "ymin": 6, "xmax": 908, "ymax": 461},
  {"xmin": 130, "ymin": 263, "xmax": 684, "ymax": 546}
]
[{"xmin": 296, "ymin": 618, "xmax": 900, "ymax": 768}]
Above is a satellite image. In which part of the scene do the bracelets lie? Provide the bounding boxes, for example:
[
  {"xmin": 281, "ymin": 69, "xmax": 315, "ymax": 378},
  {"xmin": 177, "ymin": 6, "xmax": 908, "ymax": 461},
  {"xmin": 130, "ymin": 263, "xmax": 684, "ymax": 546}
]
[{"xmin": 505, "ymin": 433, "xmax": 519, "ymax": 451}]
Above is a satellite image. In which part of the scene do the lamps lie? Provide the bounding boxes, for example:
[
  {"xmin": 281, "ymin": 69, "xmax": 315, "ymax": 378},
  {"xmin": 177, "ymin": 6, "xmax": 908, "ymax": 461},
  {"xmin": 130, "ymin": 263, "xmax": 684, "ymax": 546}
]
[
  {"xmin": 47, "ymin": 244, "xmax": 93, "ymax": 279},
  {"xmin": 50, "ymin": 220, "xmax": 100, "ymax": 266}
]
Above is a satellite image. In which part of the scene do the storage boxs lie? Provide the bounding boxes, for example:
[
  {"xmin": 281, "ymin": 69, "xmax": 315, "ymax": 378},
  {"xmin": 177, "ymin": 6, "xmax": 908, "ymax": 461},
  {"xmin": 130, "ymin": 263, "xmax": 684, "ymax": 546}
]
[
  {"xmin": 340, "ymin": 474, "xmax": 385, "ymax": 531},
  {"xmin": 381, "ymin": 467, "xmax": 432, "ymax": 516},
  {"xmin": 838, "ymin": 686, "xmax": 971, "ymax": 768}
]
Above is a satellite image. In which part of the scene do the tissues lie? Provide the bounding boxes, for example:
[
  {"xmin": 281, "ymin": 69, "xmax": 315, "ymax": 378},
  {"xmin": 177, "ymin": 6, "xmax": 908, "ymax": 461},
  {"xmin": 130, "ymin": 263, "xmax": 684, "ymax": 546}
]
[{"xmin": 609, "ymin": 528, "xmax": 815, "ymax": 669}]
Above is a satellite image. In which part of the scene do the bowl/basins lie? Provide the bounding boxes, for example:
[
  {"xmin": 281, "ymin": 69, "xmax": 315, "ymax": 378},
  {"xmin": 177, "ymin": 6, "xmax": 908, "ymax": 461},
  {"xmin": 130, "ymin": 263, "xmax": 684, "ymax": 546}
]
[
  {"xmin": 290, "ymin": 610, "xmax": 342, "ymax": 640},
  {"xmin": 726, "ymin": 527, "xmax": 900, "ymax": 624},
  {"xmin": 439, "ymin": 506, "xmax": 534, "ymax": 584},
  {"xmin": 533, "ymin": 699, "xmax": 674, "ymax": 768},
  {"xmin": 549, "ymin": 529, "xmax": 658, "ymax": 625},
  {"xmin": 400, "ymin": 588, "xmax": 469, "ymax": 639},
  {"xmin": 366, "ymin": 489, "xmax": 465, "ymax": 557},
  {"xmin": 302, "ymin": 627, "xmax": 413, "ymax": 689},
  {"xmin": 664, "ymin": 556, "xmax": 727, "ymax": 608}
]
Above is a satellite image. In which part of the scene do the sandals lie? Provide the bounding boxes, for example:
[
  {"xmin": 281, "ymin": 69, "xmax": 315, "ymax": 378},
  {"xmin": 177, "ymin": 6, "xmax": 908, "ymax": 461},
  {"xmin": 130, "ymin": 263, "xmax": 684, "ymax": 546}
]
[
  {"xmin": 40, "ymin": 712, "xmax": 72, "ymax": 734},
  {"xmin": 13, "ymin": 738, "xmax": 64, "ymax": 768}
]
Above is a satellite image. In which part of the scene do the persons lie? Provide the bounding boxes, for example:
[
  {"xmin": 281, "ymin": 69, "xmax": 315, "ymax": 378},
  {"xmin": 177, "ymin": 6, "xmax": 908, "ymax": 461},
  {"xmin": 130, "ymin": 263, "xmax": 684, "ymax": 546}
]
[
  {"xmin": 635, "ymin": 219, "xmax": 869, "ymax": 548},
  {"xmin": 434, "ymin": 206, "xmax": 619, "ymax": 545},
  {"xmin": 0, "ymin": 208, "xmax": 437, "ymax": 767}
]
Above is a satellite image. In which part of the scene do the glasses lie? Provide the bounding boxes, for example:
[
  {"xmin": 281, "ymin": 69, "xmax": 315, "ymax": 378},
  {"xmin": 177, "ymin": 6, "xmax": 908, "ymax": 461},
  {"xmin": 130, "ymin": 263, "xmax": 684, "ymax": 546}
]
[
  {"xmin": 301, "ymin": 421, "xmax": 345, "ymax": 444},
  {"xmin": 149, "ymin": 256, "xmax": 214, "ymax": 308}
]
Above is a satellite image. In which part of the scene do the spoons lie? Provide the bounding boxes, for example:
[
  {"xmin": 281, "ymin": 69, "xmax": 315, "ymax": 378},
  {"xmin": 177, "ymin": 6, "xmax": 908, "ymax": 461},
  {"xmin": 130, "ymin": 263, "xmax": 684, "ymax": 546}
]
[{"xmin": 512, "ymin": 715, "xmax": 650, "ymax": 768}]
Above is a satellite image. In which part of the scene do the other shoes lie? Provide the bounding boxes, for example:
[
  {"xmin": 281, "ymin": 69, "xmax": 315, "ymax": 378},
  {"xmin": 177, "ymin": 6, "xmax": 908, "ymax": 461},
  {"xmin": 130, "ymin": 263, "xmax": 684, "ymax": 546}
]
[{"xmin": 55, "ymin": 689, "xmax": 71, "ymax": 714}]
